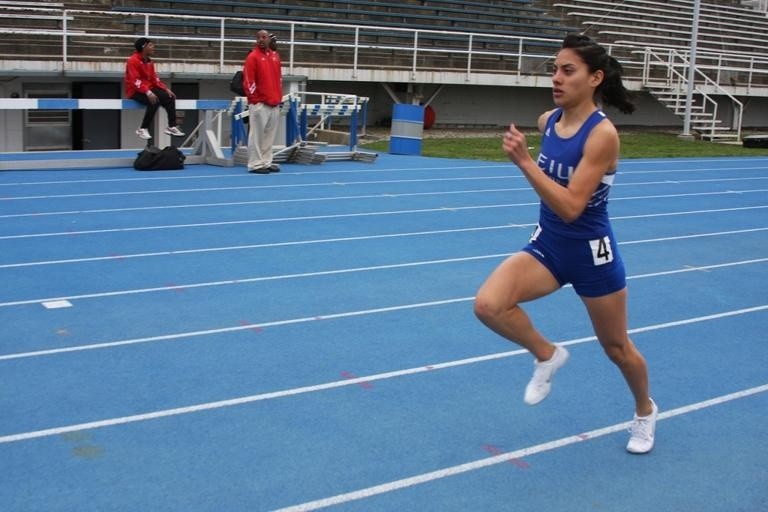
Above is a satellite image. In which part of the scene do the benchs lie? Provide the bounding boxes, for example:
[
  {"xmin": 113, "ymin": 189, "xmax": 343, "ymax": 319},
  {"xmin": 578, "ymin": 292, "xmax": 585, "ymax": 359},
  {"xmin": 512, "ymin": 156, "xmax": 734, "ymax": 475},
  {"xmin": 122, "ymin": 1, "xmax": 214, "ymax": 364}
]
[{"xmin": 1, "ymin": 0, "xmax": 768, "ymax": 88}]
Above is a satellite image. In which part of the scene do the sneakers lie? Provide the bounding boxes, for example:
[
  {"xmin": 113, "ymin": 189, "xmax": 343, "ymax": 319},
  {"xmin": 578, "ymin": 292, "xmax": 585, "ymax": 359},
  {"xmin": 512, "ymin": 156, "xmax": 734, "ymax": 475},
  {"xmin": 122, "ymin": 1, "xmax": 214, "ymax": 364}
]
[
  {"xmin": 252, "ymin": 165, "xmax": 279, "ymax": 174},
  {"xmin": 626, "ymin": 397, "xmax": 658, "ymax": 454},
  {"xmin": 135, "ymin": 128, "xmax": 152, "ymax": 139},
  {"xmin": 164, "ymin": 125, "xmax": 185, "ymax": 137},
  {"xmin": 523, "ymin": 346, "xmax": 570, "ymax": 406}
]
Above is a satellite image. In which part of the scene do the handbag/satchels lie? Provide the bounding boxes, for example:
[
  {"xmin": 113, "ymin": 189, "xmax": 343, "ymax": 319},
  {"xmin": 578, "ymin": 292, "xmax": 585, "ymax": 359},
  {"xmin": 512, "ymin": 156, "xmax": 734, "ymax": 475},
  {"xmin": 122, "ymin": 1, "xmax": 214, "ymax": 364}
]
[{"xmin": 230, "ymin": 71, "xmax": 246, "ymax": 97}]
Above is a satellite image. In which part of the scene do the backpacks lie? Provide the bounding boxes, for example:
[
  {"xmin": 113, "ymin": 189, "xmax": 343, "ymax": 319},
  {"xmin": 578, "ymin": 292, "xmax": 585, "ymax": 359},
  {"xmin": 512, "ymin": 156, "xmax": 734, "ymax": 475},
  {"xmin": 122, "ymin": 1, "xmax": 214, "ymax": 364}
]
[{"xmin": 134, "ymin": 144, "xmax": 186, "ymax": 171}]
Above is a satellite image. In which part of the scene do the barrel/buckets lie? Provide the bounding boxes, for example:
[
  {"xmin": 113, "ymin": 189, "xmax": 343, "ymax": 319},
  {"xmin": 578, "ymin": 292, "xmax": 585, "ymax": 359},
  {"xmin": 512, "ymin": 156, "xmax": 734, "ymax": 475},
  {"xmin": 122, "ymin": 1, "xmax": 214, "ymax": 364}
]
[{"xmin": 389, "ymin": 101, "xmax": 424, "ymax": 155}]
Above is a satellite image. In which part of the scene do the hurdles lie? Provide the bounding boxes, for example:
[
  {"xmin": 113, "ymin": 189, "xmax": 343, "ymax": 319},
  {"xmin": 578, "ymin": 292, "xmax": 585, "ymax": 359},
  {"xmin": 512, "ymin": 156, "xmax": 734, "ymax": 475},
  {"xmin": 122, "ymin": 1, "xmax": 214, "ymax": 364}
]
[
  {"xmin": 297, "ymin": 101, "xmax": 376, "ymax": 166},
  {"xmin": 0, "ymin": 97, "xmax": 236, "ymax": 172},
  {"xmin": 324, "ymin": 93, "xmax": 378, "ymax": 140},
  {"xmin": 228, "ymin": 93, "xmax": 316, "ymax": 168}
]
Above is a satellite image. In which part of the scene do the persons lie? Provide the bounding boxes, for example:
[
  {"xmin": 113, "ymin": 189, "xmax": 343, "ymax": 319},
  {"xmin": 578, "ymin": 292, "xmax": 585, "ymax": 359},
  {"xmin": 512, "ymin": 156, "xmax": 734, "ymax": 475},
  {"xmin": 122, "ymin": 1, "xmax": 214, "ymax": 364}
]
[
  {"xmin": 244, "ymin": 30, "xmax": 282, "ymax": 174},
  {"xmin": 124, "ymin": 38, "xmax": 186, "ymax": 139},
  {"xmin": 471, "ymin": 33, "xmax": 659, "ymax": 454},
  {"xmin": 266, "ymin": 33, "xmax": 278, "ymax": 55}
]
